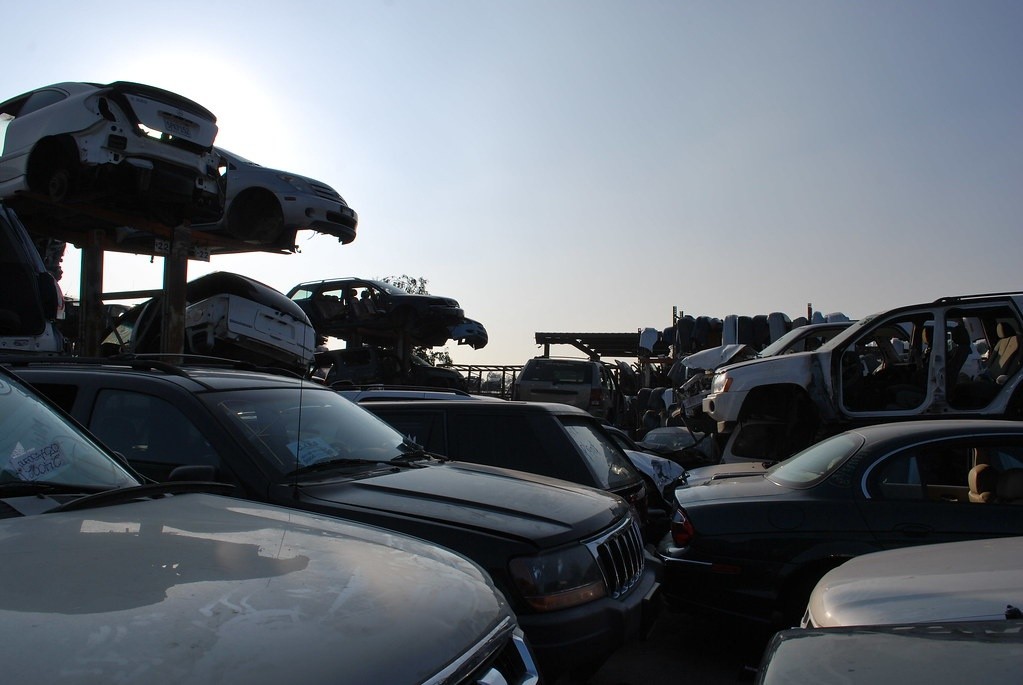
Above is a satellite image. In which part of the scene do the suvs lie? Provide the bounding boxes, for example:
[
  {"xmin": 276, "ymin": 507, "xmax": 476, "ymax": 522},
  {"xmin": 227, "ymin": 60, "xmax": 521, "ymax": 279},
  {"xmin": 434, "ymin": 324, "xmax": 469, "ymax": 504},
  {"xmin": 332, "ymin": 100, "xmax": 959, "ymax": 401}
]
[
  {"xmin": 1, "ymin": 361, "xmax": 666, "ymax": 685},
  {"xmin": 0, "ymin": 365, "xmax": 550, "ymax": 685}
]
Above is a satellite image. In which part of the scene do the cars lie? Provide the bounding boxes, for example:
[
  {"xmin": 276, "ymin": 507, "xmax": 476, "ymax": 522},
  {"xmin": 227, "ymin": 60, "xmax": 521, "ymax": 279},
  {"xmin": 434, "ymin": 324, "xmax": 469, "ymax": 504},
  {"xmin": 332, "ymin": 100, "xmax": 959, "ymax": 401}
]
[
  {"xmin": 792, "ymin": 532, "xmax": 1023, "ymax": 629},
  {"xmin": 752, "ymin": 613, "xmax": 1023, "ymax": 685},
  {"xmin": 660, "ymin": 417, "xmax": 1023, "ymax": 627},
  {"xmin": 1, "ymin": 78, "xmax": 1023, "ymax": 553}
]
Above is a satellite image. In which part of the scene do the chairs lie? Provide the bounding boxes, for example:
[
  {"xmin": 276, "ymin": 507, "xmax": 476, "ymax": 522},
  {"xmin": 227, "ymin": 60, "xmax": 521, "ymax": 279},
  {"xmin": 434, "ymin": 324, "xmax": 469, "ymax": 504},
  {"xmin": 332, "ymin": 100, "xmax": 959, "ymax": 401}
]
[
  {"xmin": 982, "ymin": 321, "xmax": 1016, "ymax": 373},
  {"xmin": 984, "ymin": 333, "xmax": 1023, "ymax": 376},
  {"xmin": 324, "ymin": 295, "xmax": 332, "ymax": 305},
  {"xmin": 332, "ymin": 296, "xmax": 341, "ymax": 305},
  {"xmin": 352, "ymin": 289, "xmax": 360, "ymax": 303},
  {"xmin": 888, "ymin": 324, "xmax": 936, "ymax": 384},
  {"xmin": 989, "ymin": 467, "xmax": 1023, "ymax": 504},
  {"xmin": 360, "ymin": 291, "xmax": 371, "ymax": 303},
  {"xmin": 804, "ymin": 337, "xmax": 822, "ymax": 351},
  {"xmin": 955, "ymin": 463, "xmax": 1000, "ymax": 504}
]
